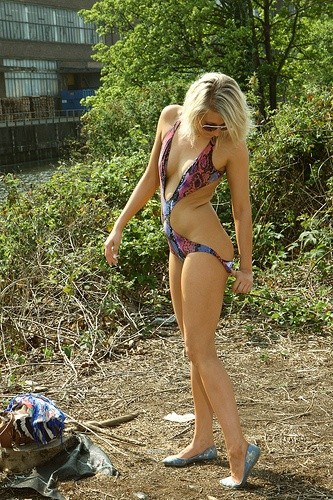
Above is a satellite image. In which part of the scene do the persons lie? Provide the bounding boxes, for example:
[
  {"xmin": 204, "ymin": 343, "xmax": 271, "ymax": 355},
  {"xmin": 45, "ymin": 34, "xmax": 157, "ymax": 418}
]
[{"xmin": 103, "ymin": 73, "xmax": 262, "ymax": 489}]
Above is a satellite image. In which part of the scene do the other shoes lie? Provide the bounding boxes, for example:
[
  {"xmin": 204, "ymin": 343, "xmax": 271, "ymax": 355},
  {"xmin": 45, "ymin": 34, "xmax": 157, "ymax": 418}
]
[
  {"xmin": 162, "ymin": 445, "xmax": 217, "ymax": 468},
  {"xmin": 219, "ymin": 444, "xmax": 261, "ymax": 490}
]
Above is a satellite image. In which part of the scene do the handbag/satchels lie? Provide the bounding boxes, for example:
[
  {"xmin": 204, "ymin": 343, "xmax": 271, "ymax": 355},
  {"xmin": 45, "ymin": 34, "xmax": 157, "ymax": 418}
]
[{"xmin": 0, "ymin": 393, "xmax": 67, "ymax": 448}]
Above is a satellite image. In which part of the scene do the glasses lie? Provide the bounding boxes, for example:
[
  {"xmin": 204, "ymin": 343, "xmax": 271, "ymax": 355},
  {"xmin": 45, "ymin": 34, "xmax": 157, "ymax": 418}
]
[{"xmin": 198, "ymin": 117, "xmax": 229, "ymax": 132}]
